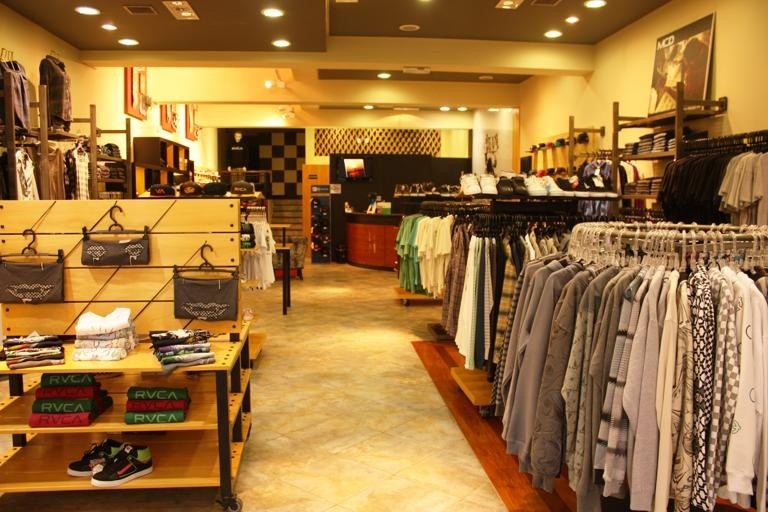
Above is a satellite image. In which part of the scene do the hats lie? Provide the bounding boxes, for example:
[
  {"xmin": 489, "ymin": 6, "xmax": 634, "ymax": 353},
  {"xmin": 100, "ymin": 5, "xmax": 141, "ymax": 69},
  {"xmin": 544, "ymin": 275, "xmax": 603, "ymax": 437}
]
[
  {"xmin": 577, "ymin": 134, "xmax": 589, "ymax": 143},
  {"xmin": 203, "ymin": 181, "xmax": 228, "ymax": 196},
  {"xmin": 535, "ymin": 170, "xmax": 546, "ymax": 176},
  {"xmin": 230, "ymin": 180, "xmax": 254, "ymax": 194},
  {"xmin": 552, "ymin": 138, "xmax": 566, "ymax": 148},
  {"xmin": 545, "ymin": 168, "xmax": 555, "ymax": 176},
  {"xmin": 553, "ymin": 167, "xmax": 565, "ymax": 175},
  {"xmin": 527, "ymin": 145, "xmax": 536, "ymax": 152},
  {"xmin": 149, "ymin": 184, "xmax": 176, "ymax": 196},
  {"xmin": 564, "ymin": 137, "xmax": 577, "ymax": 145},
  {"xmin": 535, "ymin": 143, "xmax": 546, "ymax": 151},
  {"xmin": 565, "ymin": 166, "xmax": 577, "ymax": 174},
  {"xmin": 179, "ymin": 182, "xmax": 202, "ymax": 195},
  {"xmin": 544, "ymin": 142, "xmax": 554, "ymax": 149}
]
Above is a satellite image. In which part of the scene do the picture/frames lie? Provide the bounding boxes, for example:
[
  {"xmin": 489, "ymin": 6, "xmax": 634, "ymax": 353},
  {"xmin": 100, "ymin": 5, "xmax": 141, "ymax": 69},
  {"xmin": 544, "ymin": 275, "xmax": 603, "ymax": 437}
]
[{"xmin": 648, "ymin": 11, "xmax": 716, "ymax": 117}]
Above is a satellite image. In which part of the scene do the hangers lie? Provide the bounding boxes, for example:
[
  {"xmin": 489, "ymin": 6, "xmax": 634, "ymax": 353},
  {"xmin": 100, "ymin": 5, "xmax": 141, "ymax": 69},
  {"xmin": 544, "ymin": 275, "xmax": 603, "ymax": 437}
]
[
  {"xmin": 682, "ymin": 129, "xmax": 768, "ymax": 158},
  {"xmin": 81, "ymin": 206, "xmax": 149, "ymax": 241},
  {"xmin": 0, "ymin": 48, "xmax": 21, "ymax": 71},
  {"xmin": 172, "ymin": 245, "xmax": 239, "ymax": 280},
  {"xmin": 15, "ymin": 136, "xmax": 58, "ymax": 155},
  {"xmin": 240, "ymin": 206, "xmax": 267, "ymax": 224},
  {"xmin": 0, "ymin": 229, "xmax": 64, "ymax": 264},
  {"xmin": 583, "ymin": 149, "xmax": 624, "ymax": 165},
  {"xmin": 417, "ymin": 203, "xmax": 490, "ymax": 225},
  {"xmin": 472, "ymin": 213, "xmax": 616, "ymax": 242},
  {"xmin": 45, "ymin": 48, "xmax": 62, "ymax": 63},
  {"xmin": 566, "ymin": 220, "xmax": 768, "ymax": 274},
  {"xmin": 619, "ymin": 207, "xmax": 665, "ymax": 221}
]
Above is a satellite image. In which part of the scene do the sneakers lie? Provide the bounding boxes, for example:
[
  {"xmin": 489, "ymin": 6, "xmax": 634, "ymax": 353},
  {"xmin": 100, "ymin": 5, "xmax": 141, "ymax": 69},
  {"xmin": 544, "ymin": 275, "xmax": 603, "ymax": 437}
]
[
  {"xmin": 541, "ymin": 174, "xmax": 565, "ymax": 197},
  {"xmin": 459, "ymin": 173, "xmax": 482, "ymax": 196},
  {"xmin": 496, "ymin": 174, "xmax": 514, "ymax": 196},
  {"xmin": 569, "ymin": 175, "xmax": 591, "ymax": 197},
  {"xmin": 429, "ymin": 183, "xmax": 440, "ymax": 196},
  {"xmin": 409, "ymin": 183, "xmax": 419, "ymax": 197},
  {"xmin": 598, "ymin": 174, "xmax": 620, "ymax": 198},
  {"xmin": 450, "ymin": 185, "xmax": 458, "ymax": 198},
  {"xmin": 478, "ymin": 173, "xmax": 499, "ymax": 196},
  {"xmin": 416, "ymin": 183, "xmax": 426, "ymax": 197},
  {"xmin": 525, "ymin": 174, "xmax": 549, "ymax": 197},
  {"xmin": 582, "ymin": 174, "xmax": 607, "ymax": 197},
  {"xmin": 422, "ymin": 181, "xmax": 433, "ymax": 196},
  {"xmin": 90, "ymin": 442, "xmax": 155, "ymax": 488},
  {"xmin": 555, "ymin": 176, "xmax": 576, "ymax": 197},
  {"xmin": 510, "ymin": 175, "xmax": 529, "ymax": 196},
  {"xmin": 67, "ymin": 438, "xmax": 125, "ymax": 478},
  {"xmin": 437, "ymin": 184, "xmax": 450, "ymax": 197},
  {"xmin": 394, "ymin": 184, "xmax": 403, "ymax": 197},
  {"xmin": 401, "ymin": 184, "xmax": 410, "ymax": 198}
]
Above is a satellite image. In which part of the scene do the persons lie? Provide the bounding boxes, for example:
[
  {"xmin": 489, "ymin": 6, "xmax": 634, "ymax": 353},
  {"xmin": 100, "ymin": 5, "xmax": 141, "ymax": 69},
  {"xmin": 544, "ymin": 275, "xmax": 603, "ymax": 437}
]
[
  {"xmin": 662, "ymin": 38, "xmax": 708, "ymax": 102},
  {"xmin": 226, "ymin": 131, "xmax": 249, "ymax": 185}
]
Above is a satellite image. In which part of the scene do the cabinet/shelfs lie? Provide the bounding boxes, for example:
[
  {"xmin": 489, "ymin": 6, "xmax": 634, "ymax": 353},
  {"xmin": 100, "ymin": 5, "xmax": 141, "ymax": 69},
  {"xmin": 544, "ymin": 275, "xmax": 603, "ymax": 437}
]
[
  {"xmin": 269, "ymin": 223, "xmax": 295, "ymax": 315},
  {"xmin": 612, "ymin": 82, "xmax": 727, "ymax": 200},
  {"xmin": 96, "ymin": 154, "xmax": 134, "ymax": 197},
  {"xmin": 133, "ymin": 137, "xmax": 189, "ymax": 173},
  {"xmin": 0, "ymin": 312, "xmax": 254, "ymax": 512}
]
